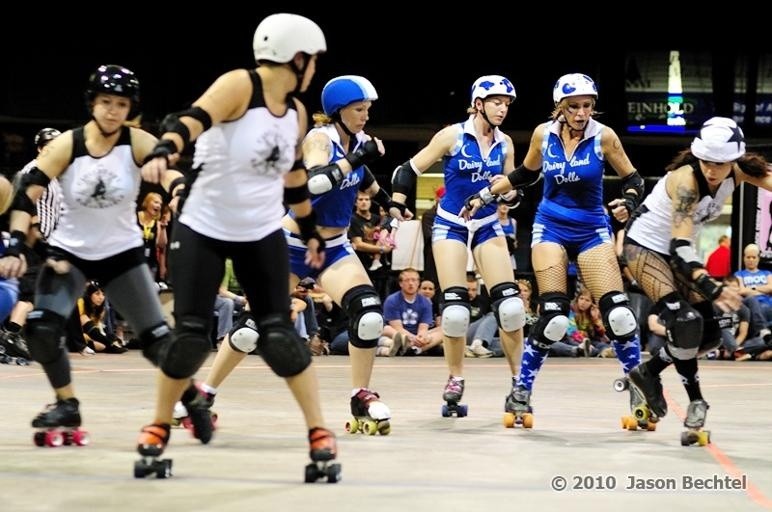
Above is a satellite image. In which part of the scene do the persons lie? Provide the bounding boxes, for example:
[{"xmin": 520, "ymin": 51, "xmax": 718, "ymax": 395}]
[
  {"xmin": 1, "ymin": 177, "xmax": 772, "ymax": 367},
  {"xmin": 388, "ymin": 73, "xmax": 530, "ymax": 417},
  {"xmin": 612, "ymin": 117, "xmax": 771, "ymax": 434},
  {"xmin": 2, "ymin": 64, "xmax": 215, "ymax": 443},
  {"xmin": 163, "ymin": 75, "xmax": 415, "ymax": 426},
  {"xmin": 500, "ymin": 73, "xmax": 660, "ymax": 431},
  {"xmin": 2, "ymin": 127, "xmax": 77, "ymax": 367},
  {"xmin": 134, "ymin": 13, "xmax": 343, "ymax": 463}
]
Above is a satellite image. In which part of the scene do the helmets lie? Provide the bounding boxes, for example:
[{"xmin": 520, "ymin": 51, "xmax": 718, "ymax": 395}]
[
  {"xmin": 253, "ymin": 12, "xmax": 329, "ymax": 66},
  {"xmin": 688, "ymin": 116, "xmax": 746, "ymax": 164},
  {"xmin": 470, "ymin": 72, "xmax": 517, "ymax": 109},
  {"xmin": 35, "ymin": 128, "xmax": 62, "ymax": 150},
  {"xmin": 90, "ymin": 65, "xmax": 140, "ymax": 113},
  {"xmin": 321, "ymin": 74, "xmax": 379, "ymax": 118},
  {"xmin": 553, "ymin": 73, "xmax": 598, "ymax": 106}
]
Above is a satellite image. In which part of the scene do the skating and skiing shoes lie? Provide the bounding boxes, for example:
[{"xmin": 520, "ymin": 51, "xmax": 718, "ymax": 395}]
[
  {"xmin": 343, "ymin": 390, "xmax": 392, "ymax": 436},
  {"xmin": 621, "ymin": 383, "xmax": 658, "ymax": 433},
  {"xmin": 679, "ymin": 399, "xmax": 713, "ymax": 447},
  {"xmin": 133, "ymin": 422, "xmax": 174, "ymax": 481},
  {"xmin": 168, "ymin": 387, "xmax": 221, "ymax": 446},
  {"xmin": 440, "ymin": 374, "xmax": 469, "ymax": 418},
  {"xmin": 302, "ymin": 427, "xmax": 343, "ymax": 486},
  {"xmin": 503, "ymin": 376, "xmax": 535, "ymax": 431},
  {"xmin": 1, "ymin": 324, "xmax": 38, "ymax": 367},
  {"xmin": 31, "ymin": 397, "xmax": 90, "ymax": 448}
]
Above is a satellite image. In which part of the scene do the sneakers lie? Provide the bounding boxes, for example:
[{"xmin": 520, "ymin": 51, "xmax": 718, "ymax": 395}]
[
  {"xmin": 369, "ymin": 260, "xmax": 384, "ymax": 271},
  {"xmin": 571, "ymin": 337, "xmax": 617, "ymax": 359},
  {"xmin": 390, "ymin": 329, "xmax": 423, "ymax": 357},
  {"xmin": 307, "ymin": 340, "xmax": 330, "ymax": 356},
  {"xmin": 464, "ymin": 342, "xmax": 496, "ymax": 359},
  {"xmin": 705, "ymin": 346, "xmax": 772, "ymax": 361},
  {"xmin": 613, "ymin": 362, "xmax": 668, "ymax": 418}
]
